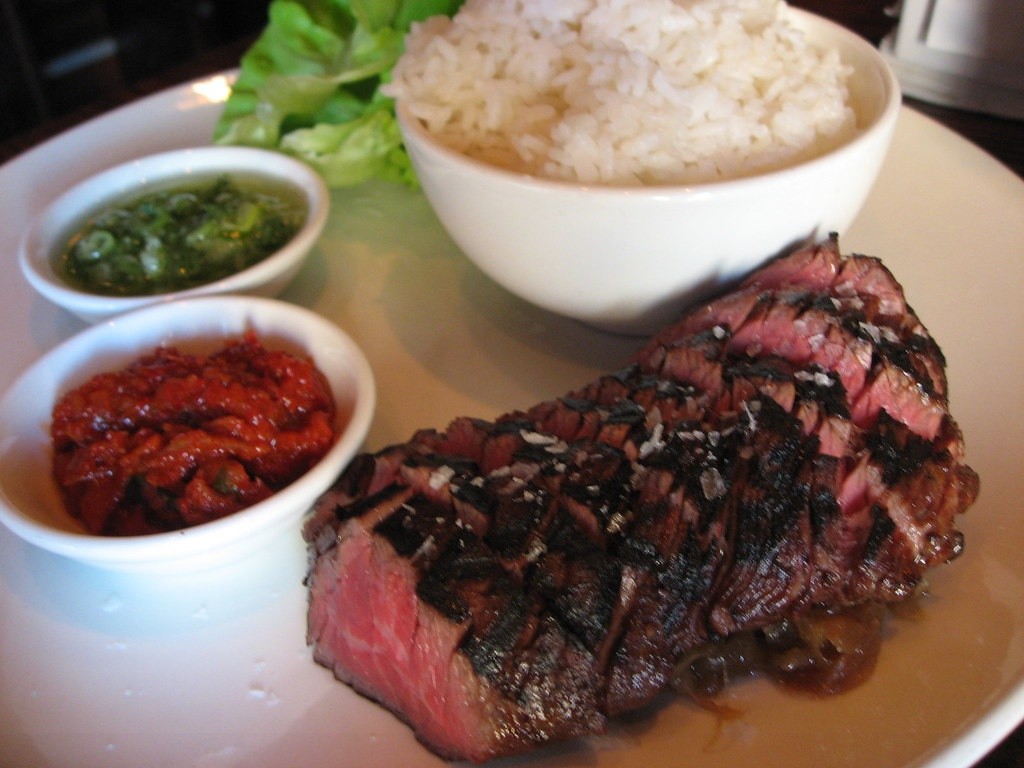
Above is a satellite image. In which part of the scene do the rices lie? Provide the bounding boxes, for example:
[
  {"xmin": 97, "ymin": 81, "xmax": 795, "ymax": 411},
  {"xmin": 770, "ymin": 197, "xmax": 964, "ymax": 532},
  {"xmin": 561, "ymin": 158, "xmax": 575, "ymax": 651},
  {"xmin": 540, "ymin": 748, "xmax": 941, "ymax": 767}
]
[{"xmin": 382, "ymin": 1, "xmax": 860, "ymax": 187}]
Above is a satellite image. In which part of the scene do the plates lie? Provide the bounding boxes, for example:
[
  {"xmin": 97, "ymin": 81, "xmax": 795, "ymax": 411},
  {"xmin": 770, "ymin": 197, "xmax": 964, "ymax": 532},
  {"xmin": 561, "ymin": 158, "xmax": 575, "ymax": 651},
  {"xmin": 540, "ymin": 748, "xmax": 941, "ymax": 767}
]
[
  {"xmin": 0, "ymin": 71, "xmax": 1024, "ymax": 768},
  {"xmin": 15, "ymin": 148, "xmax": 329, "ymax": 321},
  {"xmin": 0, "ymin": 298, "xmax": 374, "ymax": 557}
]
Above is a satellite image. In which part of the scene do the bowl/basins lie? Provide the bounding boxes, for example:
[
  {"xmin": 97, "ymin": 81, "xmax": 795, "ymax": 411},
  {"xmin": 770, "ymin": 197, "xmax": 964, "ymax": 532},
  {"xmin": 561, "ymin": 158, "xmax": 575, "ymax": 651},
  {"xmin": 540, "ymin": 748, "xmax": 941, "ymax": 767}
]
[{"xmin": 397, "ymin": 1, "xmax": 901, "ymax": 334}]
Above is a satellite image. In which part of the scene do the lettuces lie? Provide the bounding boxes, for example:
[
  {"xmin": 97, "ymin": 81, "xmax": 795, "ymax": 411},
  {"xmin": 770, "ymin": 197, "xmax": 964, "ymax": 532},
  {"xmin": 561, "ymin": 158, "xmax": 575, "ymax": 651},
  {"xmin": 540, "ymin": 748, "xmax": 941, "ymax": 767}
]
[{"xmin": 215, "ymin": 0, "xmax": 459, "ymax": 191}]
